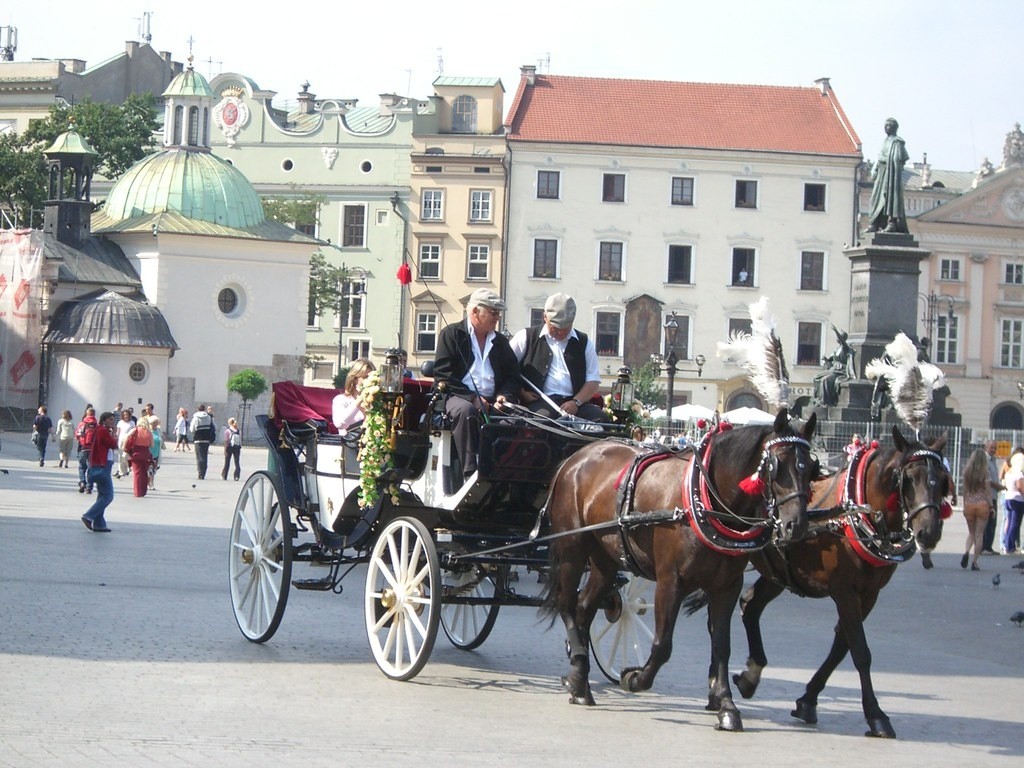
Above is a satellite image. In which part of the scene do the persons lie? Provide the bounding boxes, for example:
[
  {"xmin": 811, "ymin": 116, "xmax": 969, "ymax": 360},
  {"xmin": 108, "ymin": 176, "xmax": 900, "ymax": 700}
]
[
  {"xmin": 918, "ymin": 337, "xmax": 954, "ymax": 412},
  {"xmin": 632, "ymin": 426, "xmax": 696, "ymax": 450},
  {"xmin": 332, "ymin": 348, "xmax": 416, "ymax": 460},
  {"xmin": 112, "ymin": 402, "xmax": 166, "ymax": 497},
  {"xmin": 976, "ymin": 122, "xmax": 1024, "ymax": 178},
  {"xmin": 961, "ymin": 439, "xmax": 1024, "ymax": 570},
  {"xmin": 74, "ymin": 404, "xmax": 119, "ymax": 532},
  {"xmin": 864, "ymin": 117, "xmax": 910, "ymax": 234},
  {"xmin": 812, "ymin": 323, "xmax": 856, "ymax": 408},
  {"xmin": 173, "ymin": 408, "xmax": 194, "ymax": 453},
  {"xmin": 433, "ymin": 288, "xmax": 520, "ymax": 484},
  {"xmin": 509, "ymin": 293, "xmax": 611, "ymax": 432},
  {"xmin": 221, "ymin": 418, "xmax": 241, "ymax": 481},
  {"xmin": 33, "ymin": 406, "xmax": 74, "ymax": 468},
  {"xmin": 190, "ymin": 405, "xmax": 216, "ymax": 479},
  {"xmin": 911, "ymin": 437, "xmax": 957, "ymax": 569}
]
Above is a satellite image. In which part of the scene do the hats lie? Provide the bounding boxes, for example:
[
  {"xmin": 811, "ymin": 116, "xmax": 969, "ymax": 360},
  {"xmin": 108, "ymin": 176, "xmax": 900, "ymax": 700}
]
[
  {"xmin": 544, "ymin": 293, "xmax": 576, "ymax": 329},
  {"xmin": 471, "ymin": 287, "xmax": 507, "ymax": 311}
]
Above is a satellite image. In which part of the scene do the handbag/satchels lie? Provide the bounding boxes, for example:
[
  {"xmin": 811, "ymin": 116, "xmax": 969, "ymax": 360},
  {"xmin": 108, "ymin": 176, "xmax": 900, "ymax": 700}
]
[
  {"xmin": 125, "ymin": 427, "xmax": 139, "ymax": 453},
  {"xmin": 86, "ymin": 466, "xmax": 105, "ymax": 483},
  {"xmin": 341, "ymin": 421, "xmax": 366, "ymax": 448}
]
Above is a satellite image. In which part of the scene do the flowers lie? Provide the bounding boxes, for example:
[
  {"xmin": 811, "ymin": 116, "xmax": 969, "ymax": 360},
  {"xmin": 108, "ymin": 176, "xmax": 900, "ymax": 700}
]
[
  {"xmin": 356, "ymin": 370, "xmax": 402, "ymax": 512},
  {"xmin": 604, "ymin": 393, "xmax": 651, "ymax": 431}
]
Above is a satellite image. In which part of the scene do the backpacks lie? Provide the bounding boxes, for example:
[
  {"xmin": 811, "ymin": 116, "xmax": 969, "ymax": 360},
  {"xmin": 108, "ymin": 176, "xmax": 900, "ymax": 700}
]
[
  {"xmin": 80, "ymin": 420, "xmax": 97, "ymax": 448},
  {"xmin": 228, "ymin": 429, "xmax": 243, "ymax": 449}
]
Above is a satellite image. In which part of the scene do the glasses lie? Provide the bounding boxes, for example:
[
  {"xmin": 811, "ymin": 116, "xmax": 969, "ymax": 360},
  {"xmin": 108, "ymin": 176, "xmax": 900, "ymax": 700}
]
[{"xmin": 480, "ymin": 304, "xmax": 504, "ymax": 318}]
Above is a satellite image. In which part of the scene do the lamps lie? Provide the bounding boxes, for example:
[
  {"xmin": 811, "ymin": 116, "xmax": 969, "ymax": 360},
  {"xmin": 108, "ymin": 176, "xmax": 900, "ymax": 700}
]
[
  {"xmin": 609, "ymin": 366, "xmax": 634, "ymax": 424},
  {"xmin": 380, "ymin": 346, "xmax": 403, "ymax": 440}
]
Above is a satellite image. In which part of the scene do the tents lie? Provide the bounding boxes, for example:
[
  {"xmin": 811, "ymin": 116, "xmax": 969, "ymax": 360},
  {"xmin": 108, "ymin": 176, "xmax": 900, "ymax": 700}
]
[{"xmin": 657, "ymin": 403, "xmax": 778, "ymax": 426}]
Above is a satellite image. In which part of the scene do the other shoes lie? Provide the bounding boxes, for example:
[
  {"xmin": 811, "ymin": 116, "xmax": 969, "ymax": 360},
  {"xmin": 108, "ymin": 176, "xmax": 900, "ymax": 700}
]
[
  {"xmin": 971, "ymin": 563, "xmax": 980, "ymax": 570},
  {"xmin": 80, "ymin": 483, "xmax": 85, "ymax": 493},
  {"xmin": 88, "ymin": 489, "xmax": 92, "ymax": 493},
  {"xmin": 82, "ymin": 516, "xmax": 95, "ymax": 532},
  {"xmin": 962, "ymin": 552, "xmax": 968, "ymax": 568},
  {"xmin": 59, "ymin": 459, "xmax": 64, "ymax": 467},
  {"xmin": 147, "ymin": 484, "xmax": 155, "ymax": 490},
  {"xmin": 93, "ymin": 527, "xmax": 111, "ymax": 533},
  {"xmin": 40, "ymin": 457, "xmax": 44, "ymax": 467},
  {"xmin": 66, "ymin": 464, "xmax": 69, "ymax": 469},
  {"xmin": 985, "ymin": 547, "xmax": 1002, "ymax": 555},
  {"xmin": 1005, "ymin": 549, "xmax": 1021, "ymax": 556}
]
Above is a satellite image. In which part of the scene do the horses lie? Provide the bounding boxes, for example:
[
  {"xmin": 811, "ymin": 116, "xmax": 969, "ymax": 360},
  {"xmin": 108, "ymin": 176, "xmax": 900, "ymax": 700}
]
[
  {"xmin": 677, "ymin": 424, "xmax": 951, "ymax": 741},
  {"xmin": 531, "ymin": 407, "xmax": 820, "ymax": 733}
]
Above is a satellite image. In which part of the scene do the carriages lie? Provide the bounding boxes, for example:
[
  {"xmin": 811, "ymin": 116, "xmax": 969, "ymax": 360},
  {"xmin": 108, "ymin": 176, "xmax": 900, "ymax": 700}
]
[{"xmin": 228, "ymin": 296, "xmax": 957, "ymax": 738}]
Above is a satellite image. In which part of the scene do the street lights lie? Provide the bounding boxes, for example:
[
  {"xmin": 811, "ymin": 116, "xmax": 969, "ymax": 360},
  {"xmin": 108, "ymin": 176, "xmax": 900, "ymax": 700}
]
[
  {"xmin": 649, "ymin": 310, "xmax": 707, "ymax": 436},
  {"xmin": 919, "ymin": 289, "xmax": 956, "ymax": 364}
]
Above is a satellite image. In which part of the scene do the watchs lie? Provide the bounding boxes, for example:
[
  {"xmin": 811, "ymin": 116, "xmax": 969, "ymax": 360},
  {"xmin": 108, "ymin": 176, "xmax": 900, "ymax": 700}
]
[{"xmin": 572, "ymin": 399, "xmax": 582, "ymax": 407}]
